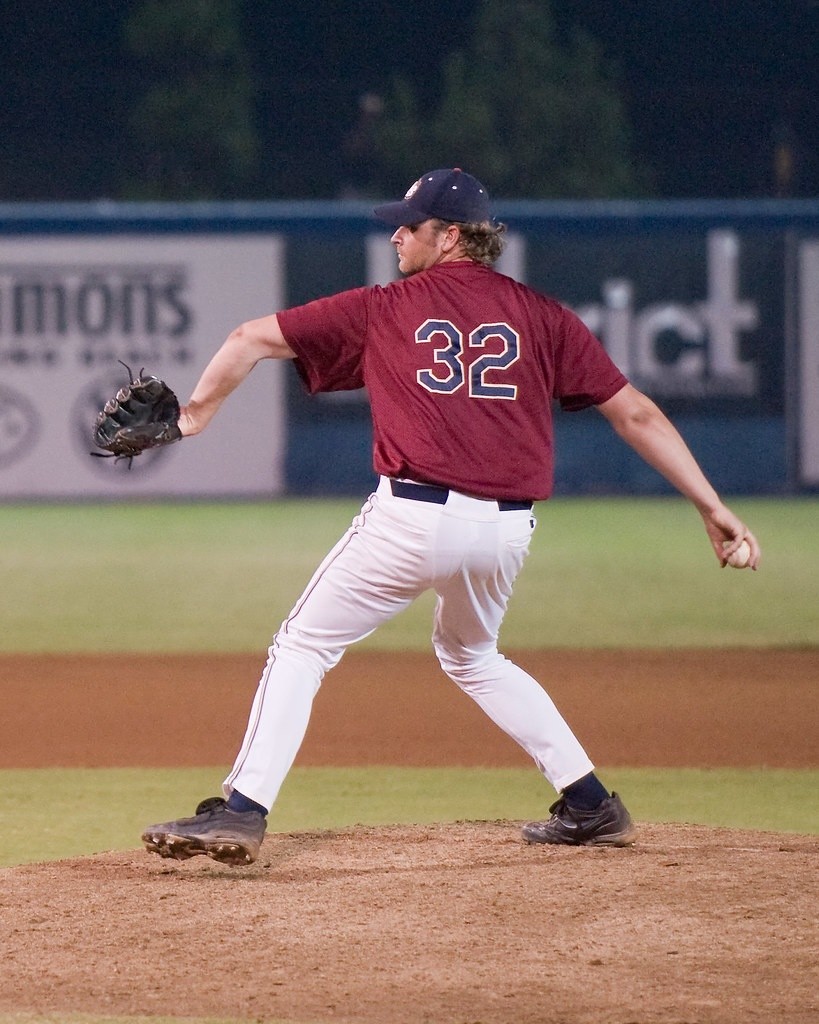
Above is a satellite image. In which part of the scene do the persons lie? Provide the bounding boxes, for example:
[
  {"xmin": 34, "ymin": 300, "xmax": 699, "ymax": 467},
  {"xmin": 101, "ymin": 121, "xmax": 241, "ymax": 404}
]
[{"xmin": 141, "ymin": 169, "xmax": 759, "ymax": 865}]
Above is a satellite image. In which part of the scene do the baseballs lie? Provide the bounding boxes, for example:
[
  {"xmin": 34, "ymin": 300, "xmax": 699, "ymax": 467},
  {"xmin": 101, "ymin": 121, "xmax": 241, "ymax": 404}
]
[{"xmin": 727, "ymin": 540, "xmax": 751, "ymax": 569}]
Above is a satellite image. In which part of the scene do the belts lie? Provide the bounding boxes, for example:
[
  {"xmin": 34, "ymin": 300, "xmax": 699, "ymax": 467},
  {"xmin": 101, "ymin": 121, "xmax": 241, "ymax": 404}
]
[{"xmin": 391, "ymin": 476, "xmax": 532, "ymax": 512}]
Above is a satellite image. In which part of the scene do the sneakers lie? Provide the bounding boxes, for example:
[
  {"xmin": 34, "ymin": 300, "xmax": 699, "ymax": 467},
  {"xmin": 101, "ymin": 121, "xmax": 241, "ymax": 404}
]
[
  {"xmin": 143, "ymin": 797, "xmax": 268, "ymax": 868},
  {"xmin": 521, "ymin": 792, "xmax": 639, "ymax": 848}
]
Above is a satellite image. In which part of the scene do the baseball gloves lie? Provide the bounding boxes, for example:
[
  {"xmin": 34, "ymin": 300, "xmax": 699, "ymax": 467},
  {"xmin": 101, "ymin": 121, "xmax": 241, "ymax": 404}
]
[{"xmin": 93, "ymin": 374, "xmax": 182, "ymax": 460}]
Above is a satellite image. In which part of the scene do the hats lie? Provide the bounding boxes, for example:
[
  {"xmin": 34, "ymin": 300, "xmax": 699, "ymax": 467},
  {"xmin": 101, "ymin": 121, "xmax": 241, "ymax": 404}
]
[{"xmin": 373, "ymin": 168, "xmax": 489, "ymax": 228}]
